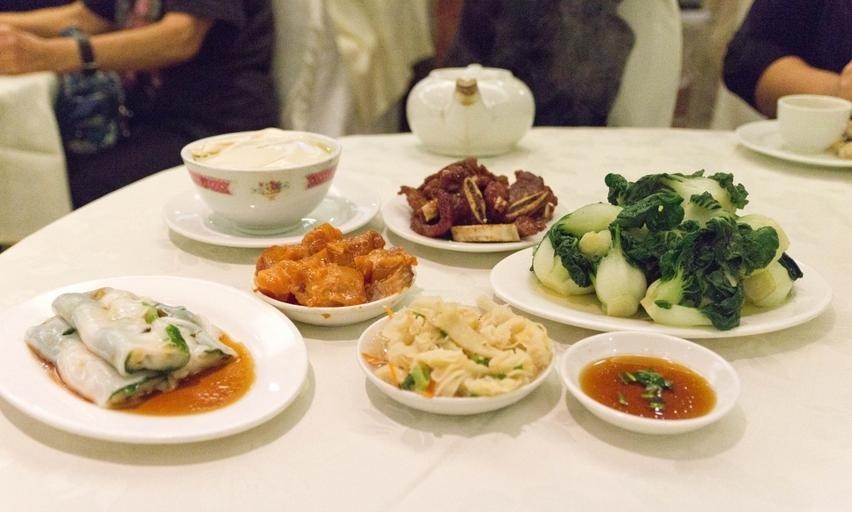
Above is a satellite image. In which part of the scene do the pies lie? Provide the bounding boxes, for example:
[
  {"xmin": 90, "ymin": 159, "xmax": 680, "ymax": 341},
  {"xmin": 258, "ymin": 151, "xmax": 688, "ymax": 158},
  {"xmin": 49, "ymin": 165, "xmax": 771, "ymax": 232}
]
[{"xmin": 25, "ymin": 285, "xmax": 234, "ymax": 408}]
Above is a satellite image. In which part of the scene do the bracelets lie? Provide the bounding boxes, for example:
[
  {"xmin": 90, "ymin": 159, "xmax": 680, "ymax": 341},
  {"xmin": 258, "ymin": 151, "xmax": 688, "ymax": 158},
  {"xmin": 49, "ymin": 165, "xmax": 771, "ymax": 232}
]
[{"xmin": 62, "ymin": 27, "xmax": 99, "ymax": 76}]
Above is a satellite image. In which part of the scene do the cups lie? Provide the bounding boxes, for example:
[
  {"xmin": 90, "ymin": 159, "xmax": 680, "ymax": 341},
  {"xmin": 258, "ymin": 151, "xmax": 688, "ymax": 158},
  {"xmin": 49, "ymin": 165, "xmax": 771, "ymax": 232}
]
[{"xmin": 776, "ymin": 94, "xmax": 852, "ymax": 155}]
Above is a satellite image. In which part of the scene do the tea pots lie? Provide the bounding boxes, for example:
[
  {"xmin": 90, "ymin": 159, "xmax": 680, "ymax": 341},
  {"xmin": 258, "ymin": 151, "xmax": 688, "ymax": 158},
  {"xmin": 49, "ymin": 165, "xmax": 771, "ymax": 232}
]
[{"xmin": 403, "ymin": 62, "xmax": 536, "ymax": 160}]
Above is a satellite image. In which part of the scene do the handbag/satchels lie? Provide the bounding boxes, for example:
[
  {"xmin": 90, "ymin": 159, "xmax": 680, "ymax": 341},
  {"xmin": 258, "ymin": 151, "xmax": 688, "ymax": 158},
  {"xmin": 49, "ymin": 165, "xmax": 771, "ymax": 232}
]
[{"xmin": 54, "ymin": 28, "xmax": 130, "ymax": 155}]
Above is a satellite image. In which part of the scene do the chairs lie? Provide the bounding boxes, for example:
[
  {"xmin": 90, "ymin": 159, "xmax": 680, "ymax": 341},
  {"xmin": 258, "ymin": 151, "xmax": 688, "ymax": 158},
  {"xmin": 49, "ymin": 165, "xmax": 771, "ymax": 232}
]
[{"xmin": 267, "ymin": 0, "xmax": 763, "ymax": 135}]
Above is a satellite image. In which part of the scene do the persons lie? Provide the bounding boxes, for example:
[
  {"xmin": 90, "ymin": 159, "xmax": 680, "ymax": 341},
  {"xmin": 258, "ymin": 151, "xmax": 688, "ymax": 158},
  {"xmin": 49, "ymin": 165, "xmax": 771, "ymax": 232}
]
[
  {"xmin": 717, "ymin": 1, "xmax": 851, "ymax": 124},
  {"xmin": 0, "ymin": 1, "xmax": 285, "ymax": 208},
  {"xmin": 401, "ymin": 0, "xmax": 635, "ymax": 132}
]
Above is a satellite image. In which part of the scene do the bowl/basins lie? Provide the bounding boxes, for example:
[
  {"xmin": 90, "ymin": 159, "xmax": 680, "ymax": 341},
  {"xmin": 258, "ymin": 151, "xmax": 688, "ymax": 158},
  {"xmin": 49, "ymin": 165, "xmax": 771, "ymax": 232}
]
[{"xmin": 178, "ymin": 128, "xmax": 345, "ymax": 238}]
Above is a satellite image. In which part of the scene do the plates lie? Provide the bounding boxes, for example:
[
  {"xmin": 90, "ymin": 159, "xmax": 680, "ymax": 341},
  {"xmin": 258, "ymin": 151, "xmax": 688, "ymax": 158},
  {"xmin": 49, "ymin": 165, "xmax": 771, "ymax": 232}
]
[
  {"xmin": 733, "ymin": 119, "xmax": 851, "ymax": 170},
  {"xmin": 0, "ymin": 272, "xmax": 308, "ymax": 446},
  {"xmin": 558, "ymin": 330, "xmax": 742, "ymax": 436},
  {"xmin": 253, "ymin": 274, "xmax": 416, "ymax": 328},
  {"xmin": 354, "ymin": 312, "xmax": 554, "ymax": 416},
  {"xmin": 490, "ymin": 243, "xmax": 835, "ymax": 340},
  {"xmin": 153, "ymin": 192, "xmax": 381, "ymax": 250},
  {"xmin": 382, "ymin": 193, "xmax": 569, "ymax": 254}
]
